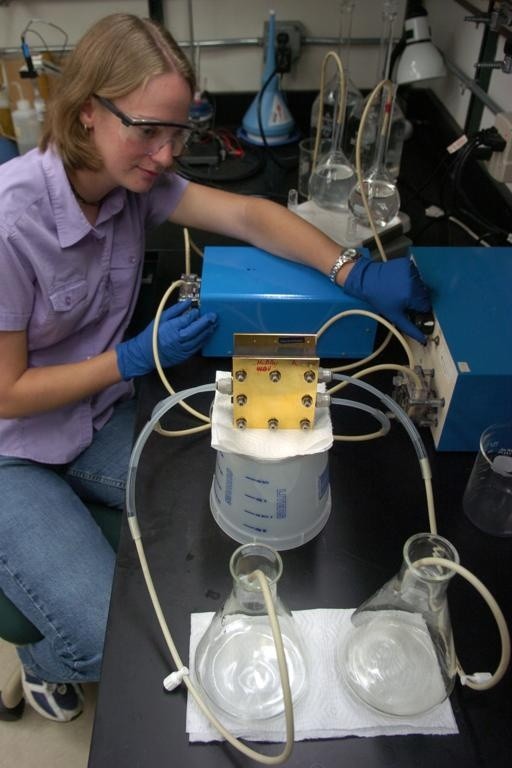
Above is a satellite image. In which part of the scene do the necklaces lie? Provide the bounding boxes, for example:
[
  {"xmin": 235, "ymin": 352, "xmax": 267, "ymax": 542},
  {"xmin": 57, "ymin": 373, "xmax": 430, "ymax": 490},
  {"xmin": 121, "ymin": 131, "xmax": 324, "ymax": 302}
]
[{"xmin": 69, "ymin": 179, "xmax": 102, "ymax": 207}]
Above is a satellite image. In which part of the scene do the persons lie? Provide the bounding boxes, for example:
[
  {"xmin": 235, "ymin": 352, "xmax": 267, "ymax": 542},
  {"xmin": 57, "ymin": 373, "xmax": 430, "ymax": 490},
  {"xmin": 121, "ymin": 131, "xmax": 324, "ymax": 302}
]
[{"xmin": 0, "ymin": 14, "xmax": 431, "ymax": 724}]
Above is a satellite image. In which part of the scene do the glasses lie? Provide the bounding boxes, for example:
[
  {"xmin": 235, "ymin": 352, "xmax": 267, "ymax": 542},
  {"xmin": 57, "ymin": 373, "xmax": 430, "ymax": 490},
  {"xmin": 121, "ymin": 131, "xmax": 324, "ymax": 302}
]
[{"xmin": 91, "ymin": 92, "xmax": 196, "ymax": 157}]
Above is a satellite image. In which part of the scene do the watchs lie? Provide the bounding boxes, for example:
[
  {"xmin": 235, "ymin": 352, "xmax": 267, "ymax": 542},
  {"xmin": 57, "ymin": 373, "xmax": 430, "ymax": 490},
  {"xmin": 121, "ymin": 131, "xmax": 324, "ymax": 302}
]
[{"xmin": 331, "ymin": 247, "xmax": 361, "ymax": 285}]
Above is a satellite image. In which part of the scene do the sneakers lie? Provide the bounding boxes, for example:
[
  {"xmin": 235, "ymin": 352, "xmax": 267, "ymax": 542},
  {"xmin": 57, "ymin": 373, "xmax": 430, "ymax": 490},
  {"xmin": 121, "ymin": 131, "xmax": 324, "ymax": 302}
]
[{"xmin": 21, "ymin": 661, "xmax": 84, "ymax": 723}]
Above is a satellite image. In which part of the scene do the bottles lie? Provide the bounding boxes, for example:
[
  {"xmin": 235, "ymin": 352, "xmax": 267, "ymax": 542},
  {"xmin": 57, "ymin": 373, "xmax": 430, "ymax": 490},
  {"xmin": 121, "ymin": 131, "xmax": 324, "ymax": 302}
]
[
  {"xmin": 307, "ymin": 71, "xmax": 406, "ymax": 228},
  {"xmin": 310, "ymin": 0, "xmax": 407, "ymax": 182},
  {"xmin": 192, "ymin": 542, "xmax": 309, "ymax": 722},
  {"xmin": 334, "ymin": 533, "xmax": 458, "ymax": 719},
  {"xmin": 9, "ymin": 96, "xmax": 42, "ymax": 155}
]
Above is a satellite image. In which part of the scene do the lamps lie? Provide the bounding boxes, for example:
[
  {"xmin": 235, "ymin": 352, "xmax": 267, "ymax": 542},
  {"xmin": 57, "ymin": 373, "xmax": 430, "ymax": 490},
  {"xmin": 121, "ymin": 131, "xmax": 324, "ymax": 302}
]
[{"xmin": 380, "ymin": 7, "xmax": 446, "ymax": 143}]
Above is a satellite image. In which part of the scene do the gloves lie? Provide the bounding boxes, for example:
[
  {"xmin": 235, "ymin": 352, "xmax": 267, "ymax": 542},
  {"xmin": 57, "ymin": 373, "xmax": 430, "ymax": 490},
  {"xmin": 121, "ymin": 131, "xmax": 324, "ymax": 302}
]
[
  {"xmin": 115, "ymin": 299, "xmax": 218, "ymax": 381},
  {"xmin": 344, "ymin": 256, "xmax": 431, "ymax": 344}
]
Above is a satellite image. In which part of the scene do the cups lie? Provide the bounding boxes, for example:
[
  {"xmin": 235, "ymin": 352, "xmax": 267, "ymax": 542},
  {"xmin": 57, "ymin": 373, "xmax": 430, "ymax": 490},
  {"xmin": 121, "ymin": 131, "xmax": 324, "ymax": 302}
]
[
  {"xmin": 295, "ymin": 135, "xmax": 346, "ymax": 204},
  {"xmin": 460, "ymin": 422, "xmax": 511, "ymax": 537}
]
